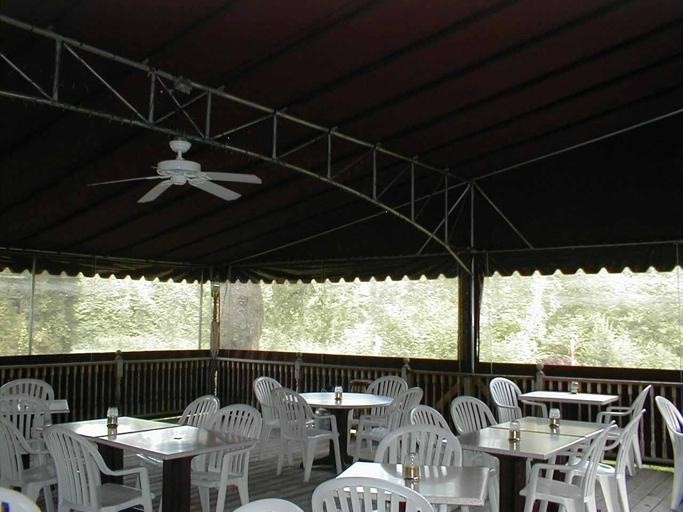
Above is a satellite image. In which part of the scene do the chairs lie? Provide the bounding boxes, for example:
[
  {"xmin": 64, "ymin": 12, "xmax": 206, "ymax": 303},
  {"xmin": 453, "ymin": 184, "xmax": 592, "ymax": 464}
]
[
  {"xmin": 655, "ymin": 395, "xmax": 683, "ymax": 512},
  {"xmin": 373, "ymin": 424, "xmax": 463, "ymax": 468},
  {"xmin": 311, "ymin": 476, "xmax": 434, "ymax": 511},
  {"xmin": 346, "ymin": 376, "xmax": 408, "ymax": 454},
  {"xmin": 490, "ymin": 376, "xmax": 549, "ymax": 421},
  {"xmin": 269, "ymin": 387, "xmax": 343, "ymax": 483},
  {"xmin": 451, "ymin": 395, "xmax": 498, "ymax": 434},
  {"xmin": 234, "ymin": 497, "xmax": 303, "ymax": 512},
  {"xmin": 137, "ymin": 394, "xmax": 263, "ymax": 511},
  {"xmin": 254, "ymin": 375, "xmax": 315, "ymax": 462},
  {"xmin": 353, "ymin": 387, "xmax": 423, "ymax": 463},
  {"xmin": 41, "ymin": 424, "xmax": 156, "ymax": 511},
  {"xmin": 518, "ymin": 429, "xmax": 608, "ymax": 511},
  {"xmin": 409, "ymin": 405, "xmax": 453, "ymax": 449},
  {"xmin": 561, "ymin": 408, "xmax": 647, "ymax": 512},
  {"xmin": 595, "ymin": 385, "xmax": 652, "ymax": 477},
  {"xmin": 2, "ymin": 375, "xmax": 72, "ymax": 512}
]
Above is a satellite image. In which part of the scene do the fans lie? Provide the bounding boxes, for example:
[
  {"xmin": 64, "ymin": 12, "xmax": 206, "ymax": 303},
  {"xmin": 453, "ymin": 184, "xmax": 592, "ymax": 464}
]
[{"xmin": 84, "ymin": 139, "xmax": 263, "ymax": 204}]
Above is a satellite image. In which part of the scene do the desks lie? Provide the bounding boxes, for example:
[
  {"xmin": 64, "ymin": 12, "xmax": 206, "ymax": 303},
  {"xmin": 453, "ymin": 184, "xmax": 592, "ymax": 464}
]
[
  {"xmin": 439, "ymin": 416, "xmax": 619, "ymax": 511},
  {"xmin": 334, "ymin": 461, "xmax": 491, "ymax": 507},
  {"xmin": 52, "ymin": 415, "xmax": 259, "ymax": 511},
  {"xmin": 282, "ymin": 393, "xmax": 394, "ymax": 470},
  {"xmin": 519, "ymin": 390, "xmax": 619, "ymax": 419}
]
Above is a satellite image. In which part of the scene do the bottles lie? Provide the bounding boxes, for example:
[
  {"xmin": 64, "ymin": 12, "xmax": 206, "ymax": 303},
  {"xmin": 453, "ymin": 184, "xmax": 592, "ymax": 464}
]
[
  {"xmin": 508, "ymin": 420, "xmax": 520, "ymax": 442},
  {"xmin": 400, "ymin": 451, "xmax": 419, "ymax": 479},
  {"xmin": 105, "ymin": 403, "xmax": 117, "ymax": 427},
  {"xmin": 333, "ymin": 385, "xmax": 342, "ymax": 400},
  {"xmin": 548, "ymin": 407, "xmax": 560, "ymax": 428},
  {"xmin": 570, "ymin": 381, "xmax": 578, "ymax": 393}
]
